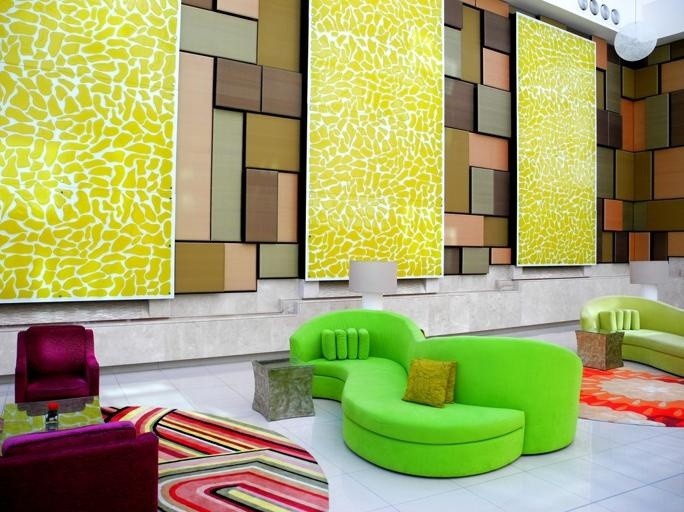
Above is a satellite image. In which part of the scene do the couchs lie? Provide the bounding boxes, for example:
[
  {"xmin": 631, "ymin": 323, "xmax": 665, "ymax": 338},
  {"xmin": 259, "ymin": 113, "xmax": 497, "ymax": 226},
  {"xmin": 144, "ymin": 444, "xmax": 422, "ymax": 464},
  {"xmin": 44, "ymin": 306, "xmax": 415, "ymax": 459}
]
[
  {"xmin": 580, "ymin": 295, "xmax": 684, "ymax": 379},
  {"xmin": 292, "ymin": 309, "xmax": 583, "ymax": 481}
]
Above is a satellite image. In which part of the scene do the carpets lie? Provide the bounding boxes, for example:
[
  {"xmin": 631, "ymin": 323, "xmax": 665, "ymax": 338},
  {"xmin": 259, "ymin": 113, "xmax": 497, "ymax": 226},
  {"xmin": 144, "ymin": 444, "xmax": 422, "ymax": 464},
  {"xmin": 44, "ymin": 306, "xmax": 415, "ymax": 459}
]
[
  {"xmin": 102, "ymin": 405, "xmax": 331, "ymax": 512},
  {"xmin": 578, "ymin": 366, "xmax": 684, "ymax": 431}
]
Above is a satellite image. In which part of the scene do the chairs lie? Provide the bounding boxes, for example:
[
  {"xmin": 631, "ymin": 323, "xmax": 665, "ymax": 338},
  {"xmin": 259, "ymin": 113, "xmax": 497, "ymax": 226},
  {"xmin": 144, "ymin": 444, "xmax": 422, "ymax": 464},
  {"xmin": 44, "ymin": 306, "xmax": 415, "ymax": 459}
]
[{"xmin": 1, "ymin": 323, "xmax": 159, "ymax": 512}]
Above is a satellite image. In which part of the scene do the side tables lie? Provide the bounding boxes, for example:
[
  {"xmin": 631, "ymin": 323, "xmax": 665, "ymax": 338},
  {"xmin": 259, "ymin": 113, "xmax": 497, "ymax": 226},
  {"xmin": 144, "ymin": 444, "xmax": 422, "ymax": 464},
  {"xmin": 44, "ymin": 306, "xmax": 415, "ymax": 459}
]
[
  {"xmin": 576, "ymin": 328, "xmax": 625, "ymax": 371},
  {"xmin": 252, "ymin": 358, "xmax": 316, "ymax": 422}
]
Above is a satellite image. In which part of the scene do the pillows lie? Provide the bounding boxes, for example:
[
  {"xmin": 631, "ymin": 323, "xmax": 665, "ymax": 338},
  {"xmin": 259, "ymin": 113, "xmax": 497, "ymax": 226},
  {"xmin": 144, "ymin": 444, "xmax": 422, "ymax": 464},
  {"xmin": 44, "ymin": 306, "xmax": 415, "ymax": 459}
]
[
  {"xmin": 323, "ymin": 328, "xmax": 371, "ymax": 361},
  {"xmin": 600, "ymin": 309, "xmax": 641, "ymax": 332},
  {"xmin": 403, "ymin": 359, "xmax": 458, "ymax": 407}
]
